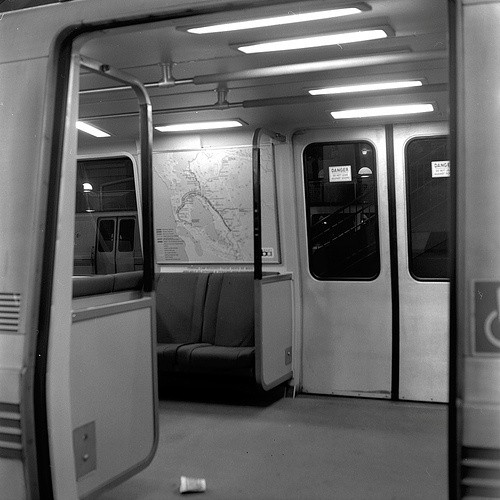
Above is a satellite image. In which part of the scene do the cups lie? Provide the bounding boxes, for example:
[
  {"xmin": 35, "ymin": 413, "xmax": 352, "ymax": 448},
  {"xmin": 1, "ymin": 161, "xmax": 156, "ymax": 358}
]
[{"xmin": 177, "ymin": 474, "xmax": 207, "ymax": 494}]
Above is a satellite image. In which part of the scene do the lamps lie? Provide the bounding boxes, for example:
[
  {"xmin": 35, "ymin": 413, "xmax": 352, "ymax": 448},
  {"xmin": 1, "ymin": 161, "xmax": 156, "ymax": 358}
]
[
  {"xmin": 174, "ymin": 2, "xmax": 373, "ymax": 35},
  {"xmin": 300, "ymin": 77, "xmax": 430, "ymax": 97},
  {"xmin": 76, "ymin": 120, "xmax": 116, "ymax": 138},
  {"xmin": 151, "ymin": 117, "xmax": 249, "ymax": 133},
  {"xmin": 82, "ymin": 183, "xmax": 94, "ymax": 192},
  {"xmin": 322, "ymin": 100, "xmax": 441, "ymax": 123},
  {"xmin": 229, "ymin": 24, "xmax": 396, "ymax": 56}
]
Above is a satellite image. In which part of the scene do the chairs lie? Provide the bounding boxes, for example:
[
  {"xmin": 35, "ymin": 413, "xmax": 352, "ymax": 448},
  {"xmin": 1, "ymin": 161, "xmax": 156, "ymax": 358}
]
[
  {"xmin": 177, "ymin": 271, "xmax": 278, "ymax": 406},
  {"xmin": 156, "ymin": 271, "xmax": 213, "ymax": 400}
]
